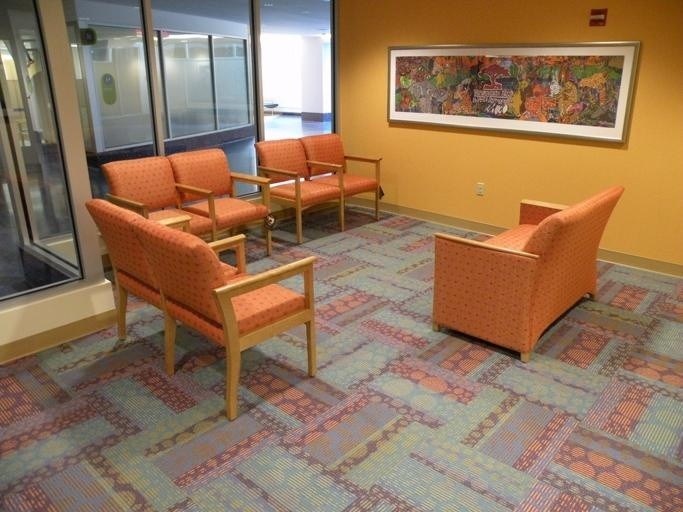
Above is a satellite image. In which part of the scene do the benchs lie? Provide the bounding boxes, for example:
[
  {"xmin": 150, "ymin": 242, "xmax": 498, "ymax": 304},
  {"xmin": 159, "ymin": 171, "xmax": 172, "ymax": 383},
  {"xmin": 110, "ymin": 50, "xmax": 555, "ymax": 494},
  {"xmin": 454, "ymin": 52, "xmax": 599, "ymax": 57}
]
[{"xmin": 431, "ymin": 184, "xmax": 626, "ymax": 364}]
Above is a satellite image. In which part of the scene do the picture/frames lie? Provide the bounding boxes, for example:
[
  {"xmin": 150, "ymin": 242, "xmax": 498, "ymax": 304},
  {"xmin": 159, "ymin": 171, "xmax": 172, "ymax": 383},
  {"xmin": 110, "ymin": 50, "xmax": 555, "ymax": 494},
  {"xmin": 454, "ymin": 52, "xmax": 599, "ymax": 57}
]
[{"xmin": 386, "ymin": 40, "xmax": 643, "ymax": 151}]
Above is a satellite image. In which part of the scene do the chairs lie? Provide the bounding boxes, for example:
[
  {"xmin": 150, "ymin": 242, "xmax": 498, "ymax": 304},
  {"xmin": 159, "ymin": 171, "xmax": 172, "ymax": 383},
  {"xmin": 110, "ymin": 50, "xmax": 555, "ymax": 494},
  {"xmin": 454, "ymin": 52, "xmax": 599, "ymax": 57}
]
[
  {"xmin": 254, "ymin": 138, "xmax": 344, "ymax": 245},
  {"xmin": 300, "ymin": 132, "xmax": 381, "ymax": 233},
  {"xmin": 99, "ymin": 152, "xmax": 220, "ymax": 261},
  {"xmin": 167, "ymin": 148, "xmax": 274, "ymax": 257},
  {"xmin": 85, "ymin": 197, "xmax": 236, "ymax": 380},
  {"xmin": 131, "ymin": 219, "xmax": 322, "ymax": 420}
]
